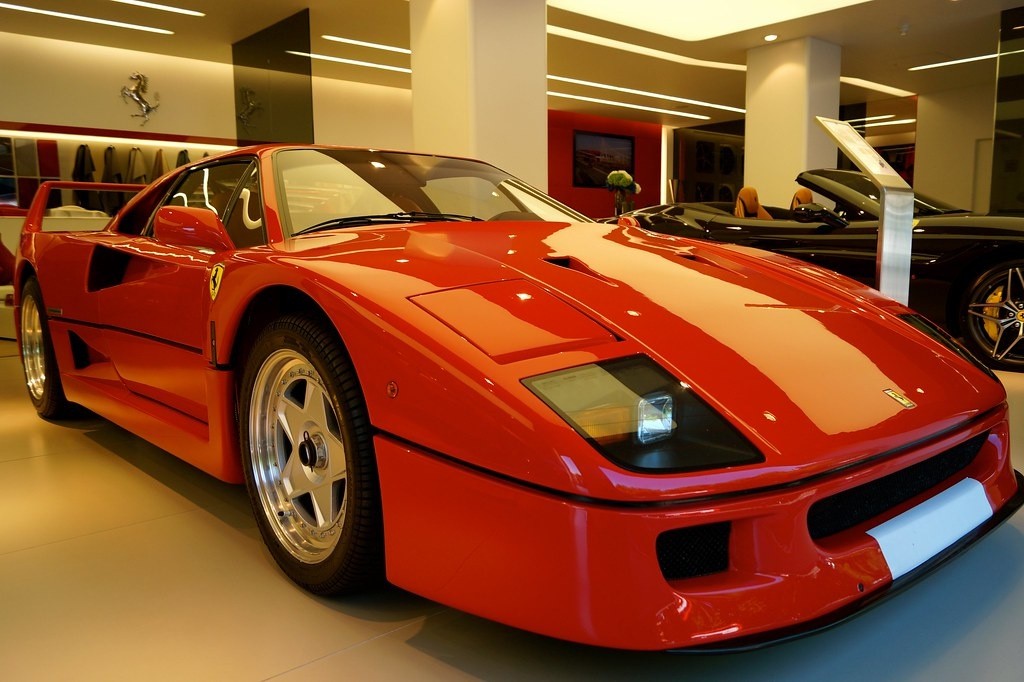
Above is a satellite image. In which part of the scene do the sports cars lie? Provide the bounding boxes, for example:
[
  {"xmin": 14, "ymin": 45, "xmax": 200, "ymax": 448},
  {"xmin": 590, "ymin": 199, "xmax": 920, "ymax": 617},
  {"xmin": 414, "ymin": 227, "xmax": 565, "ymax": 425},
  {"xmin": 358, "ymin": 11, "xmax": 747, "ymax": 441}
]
[
  {"xmin": 591, "ymin": 165, "xmax": 1024, "ymax": 373},
  {"xmin": 6, "ymin": 144, "xmax": 1024, "ymax": 656}
]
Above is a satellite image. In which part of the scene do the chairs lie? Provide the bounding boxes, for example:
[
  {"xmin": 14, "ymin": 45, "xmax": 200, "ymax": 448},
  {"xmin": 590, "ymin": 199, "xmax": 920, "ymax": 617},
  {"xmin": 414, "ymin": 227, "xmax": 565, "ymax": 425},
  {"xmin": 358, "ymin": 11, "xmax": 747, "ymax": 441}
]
[
  {"xmin": 212, "ymin": 190, "xmax": 263, "ymax": 250},
  {"xmin": 734, "ymin": 186, "xmax": 774, "ymax": 220},
  {"xmin": 787, "ymin": 188, "xmax": 814, "ymax": 220}
]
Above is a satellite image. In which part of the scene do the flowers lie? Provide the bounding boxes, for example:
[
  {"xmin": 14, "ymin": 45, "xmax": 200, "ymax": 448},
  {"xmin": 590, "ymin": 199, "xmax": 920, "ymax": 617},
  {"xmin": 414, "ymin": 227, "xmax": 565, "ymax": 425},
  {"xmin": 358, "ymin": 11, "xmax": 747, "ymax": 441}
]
[{"xmin": 604, "ymin": 170, "xmax": 641, "ymax": 194}]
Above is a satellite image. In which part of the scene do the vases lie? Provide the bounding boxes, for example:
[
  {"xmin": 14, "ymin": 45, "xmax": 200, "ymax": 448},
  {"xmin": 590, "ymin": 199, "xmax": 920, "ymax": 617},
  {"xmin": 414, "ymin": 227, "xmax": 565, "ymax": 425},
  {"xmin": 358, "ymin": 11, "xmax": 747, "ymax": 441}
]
[{"xmin": 613, "ymin": 186, "xmax": 624, "ymax": 217}]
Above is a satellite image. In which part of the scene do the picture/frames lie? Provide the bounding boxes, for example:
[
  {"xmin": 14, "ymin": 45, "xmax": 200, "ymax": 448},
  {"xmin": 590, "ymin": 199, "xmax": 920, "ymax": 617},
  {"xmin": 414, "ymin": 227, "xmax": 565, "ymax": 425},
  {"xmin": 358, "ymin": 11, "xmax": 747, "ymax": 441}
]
[{"xmin": 572, "ymin": 129, "xmax": 635, "ymax": 188}]
